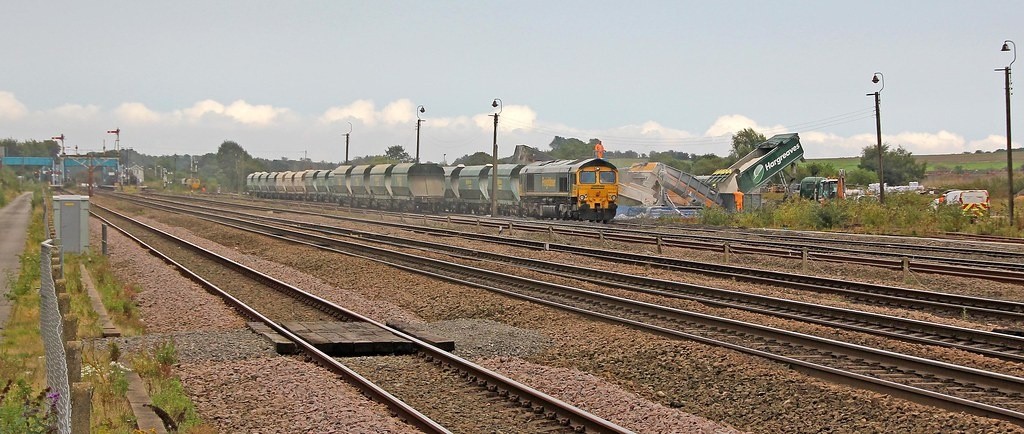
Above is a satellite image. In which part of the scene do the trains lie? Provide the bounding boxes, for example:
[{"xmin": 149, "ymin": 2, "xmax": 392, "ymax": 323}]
[
  {"xmin": 247, "ymin": 161, "xmax": 446, "ymax": 213},
  {"xmin": 442, "ymin": 150, "xmax": 619, "ymax": 224}
]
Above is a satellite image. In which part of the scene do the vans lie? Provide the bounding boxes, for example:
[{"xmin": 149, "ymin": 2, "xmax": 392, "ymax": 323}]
[{"xmin": 929, "ymin": 188, "xmax": 991, "ymax": 224}]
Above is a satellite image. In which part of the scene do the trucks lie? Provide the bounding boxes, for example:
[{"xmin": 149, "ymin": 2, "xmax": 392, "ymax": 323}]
[
  {"xmin": 726, "ymin": 133, "xmax": 839, "ymax": 203},
  {"xmin": 628, "ymin": 161, "xmax": 745, "ymax": 216}
]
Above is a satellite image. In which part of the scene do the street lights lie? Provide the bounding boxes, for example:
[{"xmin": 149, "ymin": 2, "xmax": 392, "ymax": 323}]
[
  {"xmin": 415, "ymin": 104, "xmax": 427, "ymax": 164},
  {"xmin": 341, "ymin": 120, "xmax": 352, "ymax": 164},
  {"xmin": 865, "ymin": 71, "xmax": 885, "ymax": 206},
  {"xmin": 487, "ymin": 97, "xmax": 503, "ymax": 219},
  {"xmin": 993, "ymin": 39, "xmax": 1017, "ymax": 229}
]
